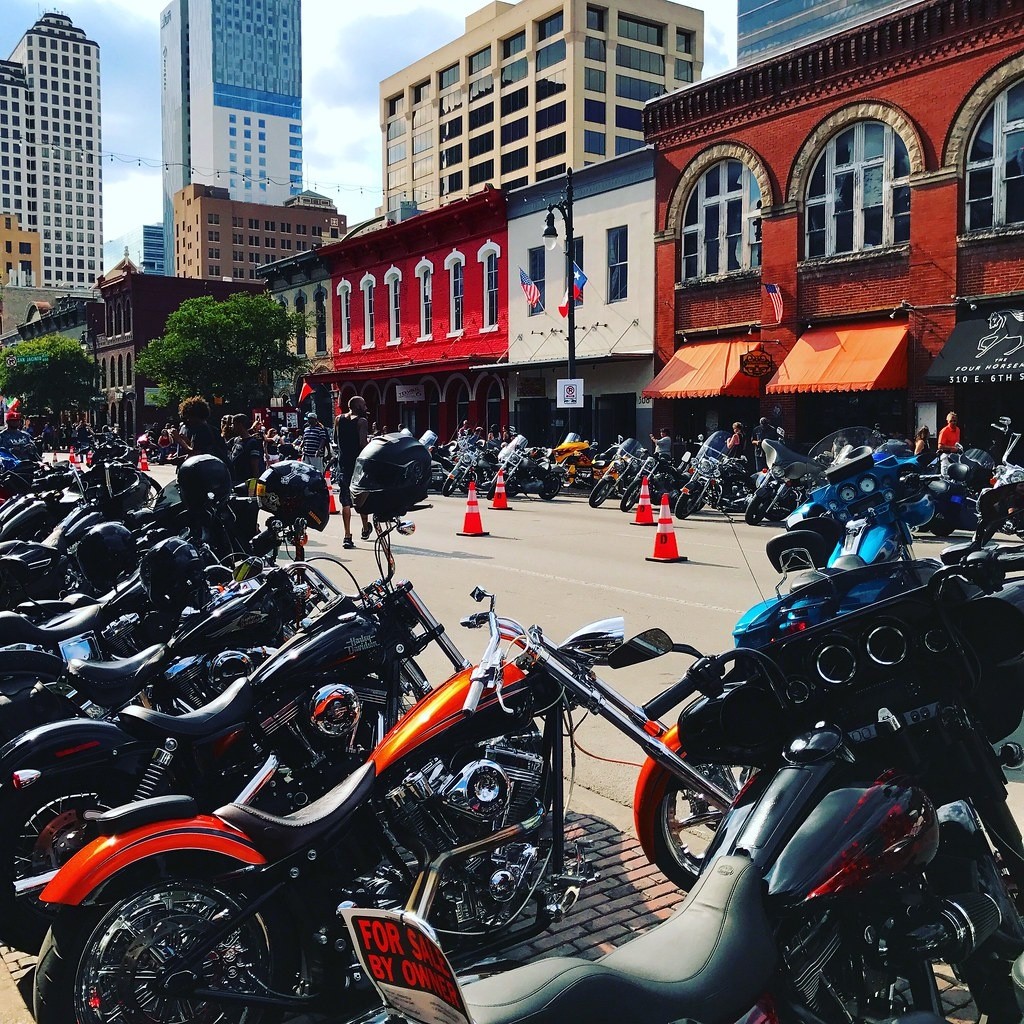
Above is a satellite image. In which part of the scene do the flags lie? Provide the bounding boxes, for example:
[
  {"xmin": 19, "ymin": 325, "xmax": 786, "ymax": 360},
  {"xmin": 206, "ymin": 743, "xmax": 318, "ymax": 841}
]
[
  {"xmin": 765, "ymin": 284, "xmax": 783, "ymax": 323},
  {"xmin": 558, "ymin": 261, "xmax": 588, "ymax": 318},
  {"xmin": 520, "ymin": 269, "xmax": 540, "ymax": 309}
]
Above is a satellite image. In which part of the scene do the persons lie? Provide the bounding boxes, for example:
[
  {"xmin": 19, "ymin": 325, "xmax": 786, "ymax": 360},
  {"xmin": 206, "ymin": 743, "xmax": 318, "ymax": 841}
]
[
  {"xmin": 649, "ymin": 427, "xmax": 671, "ymax": 458},
  {"xmin": 727, "ymin": 422, "xmax": 746, "ymax": 459},
  {"xmin": 914, "ymin": 426, "xmax": 931, "ymax": 455},
  {"xmin": 938, "ymin": 412, "xmax": 960, "ymax": 453},
  {"xmin": 751, "ymin": 417, "xmax": 777, "ymax": 472},
  {"xmin": 0, "ymin": 395, "xmax": 517, "ymax": 549}
]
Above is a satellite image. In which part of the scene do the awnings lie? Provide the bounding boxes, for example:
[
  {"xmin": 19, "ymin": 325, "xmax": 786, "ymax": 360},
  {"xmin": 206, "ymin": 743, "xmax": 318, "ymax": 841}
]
[
  {"xmin": 765, "ymin": 316, "xmax": 909, "ymax": 395},
  {"xmin": 922, "ymin": 300, "xmax": 1024, "ymax": 384},
  {"xmin": 641, "ymin": 332, "xmax": 761, "ymax": 399}
]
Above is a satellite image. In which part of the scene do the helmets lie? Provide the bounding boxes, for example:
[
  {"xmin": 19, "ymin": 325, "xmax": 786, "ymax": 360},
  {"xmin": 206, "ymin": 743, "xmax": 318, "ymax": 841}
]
[
  {"xmin": 174, "ymin": 454, "xmax": 233, "ymax": 515},
  {"xmin": 349, "ymin": 431, "xmax": 432, "ymax": 514},
  {"xmin": 76, "ymin": 522, "xmax": 138, "ymax": 595},
  {"xmin": 139, "ymin": 536, "xmax": 204, "ymax": 606},
  {"xmin": 256, "ymin": 460, "xmax": 330, "ymax": 532},
  {"xmin": 96, "ymin": 470, "xmax": 151, "ymax": 521}
]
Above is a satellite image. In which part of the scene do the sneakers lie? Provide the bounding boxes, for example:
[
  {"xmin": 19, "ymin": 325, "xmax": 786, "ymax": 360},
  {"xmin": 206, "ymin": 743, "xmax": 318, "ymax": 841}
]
[
  {"xmin": 361, "ymin": 522, "xmax": 373, "ymax": 539},
  {"xmin": 342, "ymin": 534, "xmax": 356, "ymax": 549}
]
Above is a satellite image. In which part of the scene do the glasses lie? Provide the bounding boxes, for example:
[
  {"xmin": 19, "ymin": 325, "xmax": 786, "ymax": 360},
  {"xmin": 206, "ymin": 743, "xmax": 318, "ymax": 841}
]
[{"xmin": 949, "ymin": 420, "xmax": 956, "ymax": 422}]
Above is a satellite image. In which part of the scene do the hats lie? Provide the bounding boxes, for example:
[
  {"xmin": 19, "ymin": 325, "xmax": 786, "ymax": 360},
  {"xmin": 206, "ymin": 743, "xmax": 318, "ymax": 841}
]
[
  {"xmin": 5, "ymin": 411, "xmax": 20, "ymax": 421},
  {"xmin": 305, "ymin": 413, "xmax": 318, "ymax": 420}
]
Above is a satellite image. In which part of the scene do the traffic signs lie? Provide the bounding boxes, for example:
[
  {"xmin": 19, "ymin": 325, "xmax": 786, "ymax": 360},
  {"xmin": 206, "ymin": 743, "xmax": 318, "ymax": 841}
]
[{"xmin": 89, "ymin": 396, "xmax": 106, "ymax": 402}]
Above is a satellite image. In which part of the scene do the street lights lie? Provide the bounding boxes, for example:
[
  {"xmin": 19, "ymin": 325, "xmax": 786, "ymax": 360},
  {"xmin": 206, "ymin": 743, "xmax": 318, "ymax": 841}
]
[
  {"xmin": 79, "ymin": 313, "xmax": 101, "ymax": 432},
  {"xmin": 542, "ymin": 167, "xmax": 577, "ymax": 434}
]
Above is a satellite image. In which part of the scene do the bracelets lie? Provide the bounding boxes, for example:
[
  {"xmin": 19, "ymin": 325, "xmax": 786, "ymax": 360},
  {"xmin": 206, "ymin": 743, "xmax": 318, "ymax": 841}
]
[{"xmin": 651, "ymin": 437, "xmax": 654, "ymax": 440}]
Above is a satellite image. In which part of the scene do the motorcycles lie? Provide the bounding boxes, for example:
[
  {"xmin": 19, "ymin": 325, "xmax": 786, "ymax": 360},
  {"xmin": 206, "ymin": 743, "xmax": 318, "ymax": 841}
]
[
  {"xmin": 0, "ymin": 428, "xmax": 553, "ymax": 957},
  {"xmin": 336, "ymin": 482, "xmax": 1024, "ymax": 1024},
  {"xmin": 486, "ymin": 416, "xmax": 1024, "ymax": 547},
  {"xmin": 33, "ymin": 585, "xmax": 760, "ymax": 1024},
  {"xmin": 732, "ymin": 425, "xmax": 943, "ymax": 649}
]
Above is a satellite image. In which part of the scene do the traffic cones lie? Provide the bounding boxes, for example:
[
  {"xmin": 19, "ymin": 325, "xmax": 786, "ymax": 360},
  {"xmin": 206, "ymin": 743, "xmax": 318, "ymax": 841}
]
[
  {"xmin": 645, "ymin": 493, "xmax": 687, "ymax": 563},
  {"xmin": 456, "ymin": 482, "xmax": 490, "ymax": 537},
  {"xmin": 87, "ymin": 451, "xmax": 92, "ymax": 464},
  {"xmin": 141, "ymin": 449, "xmax": 150, "ymax": 471},
  {"xmin": 53, "ymin": 451, "xmax": 58, "ymax": 463},
  {"xmin": 488, "ymin": 471, "xmax": 513, "ymax": 511},
  {"xmin": 629, "ymin": 476, "xmax": 658, "ymax": 526},
  {"xmin": 323, "ymin": 471, "xmax": 340, "ymax": 515},
  {"xmin": 73, "ymin": 456, "xmax": 81, "ymax": 470},
  {"xmin": 69, "ymin": 446, "xmax": 75, "ymax": 463},
  {"xmin": 136, "ymin": 456, "xmax": 142, "ymax": 470}
]
